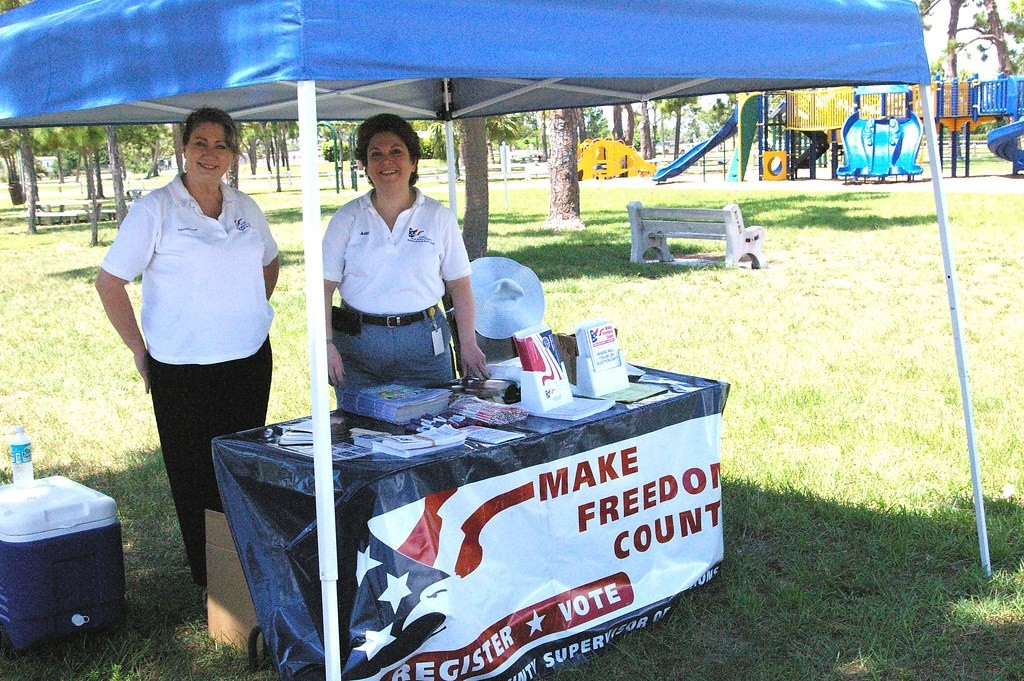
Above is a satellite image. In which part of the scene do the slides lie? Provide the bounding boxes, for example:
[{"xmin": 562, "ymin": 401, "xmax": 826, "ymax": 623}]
[
  {"xmin": 982, "ymin": 119, "xmax": 1021, "ymax": 178},
  {"xmin": 651, "ymin": 107, "xmax": 738, "ymax": 185},
  {"xmin": 893, "ymin": 112, "xmax": 926, "ymax": 183},
  {"xmin": 834, "ymin": 110, "xmax": 868, "ymax": 184},
  {"xmin": 866, "ymin": 117, "xmax": 892, "ymax": 184}
]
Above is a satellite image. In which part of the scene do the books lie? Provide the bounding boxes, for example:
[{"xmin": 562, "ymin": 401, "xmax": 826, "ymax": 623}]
[{"xmin": 276, "ymin": 382, "xmax": 527, "ymax": 461}]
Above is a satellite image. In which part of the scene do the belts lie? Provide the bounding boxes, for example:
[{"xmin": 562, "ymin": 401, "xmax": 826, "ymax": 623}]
[{"xmin": 363, "ymin": 303, "xmax": 439, "ymax": 328}]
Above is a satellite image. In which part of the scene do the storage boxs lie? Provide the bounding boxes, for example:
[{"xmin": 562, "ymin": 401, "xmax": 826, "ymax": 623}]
[
  {"xmin": 0, "ymin": 475, "xmax": 127, "ymax": 651},
  {"xmin": 204, "ymin": 507, "xmax": 266, "ymax": 661}
]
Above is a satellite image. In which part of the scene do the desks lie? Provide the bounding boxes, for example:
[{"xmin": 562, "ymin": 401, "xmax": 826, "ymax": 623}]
[
  {"xmin": 210, "ymin": 354, "xmax": 731, "ymax": 680},
  {"xmin": 35, "ymin": 199, "xmax": 110, "ymax": 223}
]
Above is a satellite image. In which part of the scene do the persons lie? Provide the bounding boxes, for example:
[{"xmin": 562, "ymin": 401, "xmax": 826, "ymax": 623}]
[
  {"xmin": 96, "ymin": 107, "xmax": 279, "ymax": 608},
  {"xmin": 324, "ymin": 113, "xmax": 491, "ymax": 408}
]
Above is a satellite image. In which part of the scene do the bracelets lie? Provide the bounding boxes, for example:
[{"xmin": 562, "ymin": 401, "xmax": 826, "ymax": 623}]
[{"xmin": 326, "ymin": 338, "xmax": 332, "ymax": 346}]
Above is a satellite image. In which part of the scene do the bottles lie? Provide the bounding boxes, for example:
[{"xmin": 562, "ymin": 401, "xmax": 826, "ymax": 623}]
[{"xmin": 9, "ymin": 426, "xmax": 36, "ymax": 488}]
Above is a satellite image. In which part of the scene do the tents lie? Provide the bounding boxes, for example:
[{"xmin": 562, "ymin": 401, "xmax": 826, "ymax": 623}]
[{"xmin": 0, "ymin": 0, "xmax": 995, "ymax": 681}]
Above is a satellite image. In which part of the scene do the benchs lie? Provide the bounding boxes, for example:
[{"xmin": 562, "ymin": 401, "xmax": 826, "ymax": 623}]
[
  {"xmin": 626, "ymin": 200, "xmax": 769, "ymax": 268},
  {"xmin": 19, "ymin": 209, "xmax": 116, "ymax": 225}
]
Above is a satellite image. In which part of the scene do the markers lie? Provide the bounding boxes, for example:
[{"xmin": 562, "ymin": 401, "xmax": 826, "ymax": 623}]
[{"xmin": 405, "ymin": 412, "xmax": 474, "ymax": 434}]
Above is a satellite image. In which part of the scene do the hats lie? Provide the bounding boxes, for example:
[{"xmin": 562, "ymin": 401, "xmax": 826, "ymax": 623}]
[{"xmin": 470, "ymin": 256, "xmax": 545, "ymax": 339}]
